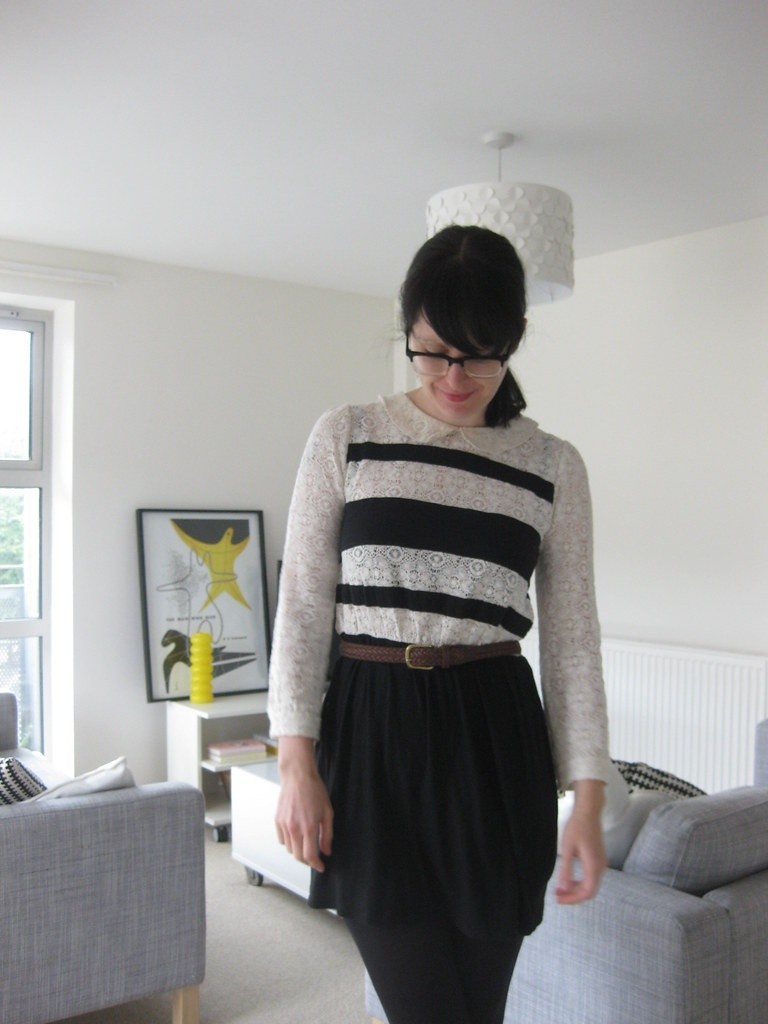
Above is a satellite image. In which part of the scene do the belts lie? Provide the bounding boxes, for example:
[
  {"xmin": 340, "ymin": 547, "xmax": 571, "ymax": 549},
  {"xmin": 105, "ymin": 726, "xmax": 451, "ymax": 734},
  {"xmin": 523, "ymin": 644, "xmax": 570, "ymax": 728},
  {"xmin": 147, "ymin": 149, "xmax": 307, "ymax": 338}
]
[{"xmin": 338, "ymin": 640, "xmax": 522, "ymax": 670}]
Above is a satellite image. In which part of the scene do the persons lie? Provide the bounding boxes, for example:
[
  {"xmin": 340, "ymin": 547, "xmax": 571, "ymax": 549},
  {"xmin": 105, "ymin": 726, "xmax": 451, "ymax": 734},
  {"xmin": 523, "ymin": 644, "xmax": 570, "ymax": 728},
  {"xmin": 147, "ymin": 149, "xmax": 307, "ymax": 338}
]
[{"xmin": 266, "ymin": 224, "xmax": 610, "ymax": 1024}]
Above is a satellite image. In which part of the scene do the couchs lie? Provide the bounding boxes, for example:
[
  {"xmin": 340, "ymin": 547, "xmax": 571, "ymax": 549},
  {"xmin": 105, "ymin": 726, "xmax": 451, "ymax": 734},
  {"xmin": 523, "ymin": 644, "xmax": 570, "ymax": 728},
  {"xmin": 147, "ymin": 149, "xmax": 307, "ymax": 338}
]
[
  {"xmin": 0, "ymin": 692, "xmax": 205, "ymax": 1024},
  {"xmin": 359, "ymin": 718, "xmax": 767, "ymax": 1024}
]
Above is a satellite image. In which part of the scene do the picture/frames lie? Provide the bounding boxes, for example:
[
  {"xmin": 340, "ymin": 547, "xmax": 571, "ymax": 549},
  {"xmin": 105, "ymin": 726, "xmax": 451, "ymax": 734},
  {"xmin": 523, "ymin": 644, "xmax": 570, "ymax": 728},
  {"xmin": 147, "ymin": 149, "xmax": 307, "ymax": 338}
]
[{"xmin": 135, "ymin": 508, "xmax": 270, "ymax": 704}]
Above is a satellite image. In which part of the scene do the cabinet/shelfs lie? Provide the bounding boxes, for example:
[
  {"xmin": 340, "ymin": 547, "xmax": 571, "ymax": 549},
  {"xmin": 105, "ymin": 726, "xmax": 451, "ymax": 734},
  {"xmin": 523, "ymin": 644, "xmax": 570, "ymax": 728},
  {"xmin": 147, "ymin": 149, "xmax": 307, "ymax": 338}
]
[{"xmin": 165, "ymin": 693, "xmax": 279, "ymax": 842}]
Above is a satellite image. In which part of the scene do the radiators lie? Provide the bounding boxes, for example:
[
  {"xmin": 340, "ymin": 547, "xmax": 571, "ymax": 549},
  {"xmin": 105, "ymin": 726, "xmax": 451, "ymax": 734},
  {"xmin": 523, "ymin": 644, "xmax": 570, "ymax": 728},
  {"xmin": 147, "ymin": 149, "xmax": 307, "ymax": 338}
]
[{"xmin": 518, "ymin": 628, "xmax": 767, "ymax": 796}]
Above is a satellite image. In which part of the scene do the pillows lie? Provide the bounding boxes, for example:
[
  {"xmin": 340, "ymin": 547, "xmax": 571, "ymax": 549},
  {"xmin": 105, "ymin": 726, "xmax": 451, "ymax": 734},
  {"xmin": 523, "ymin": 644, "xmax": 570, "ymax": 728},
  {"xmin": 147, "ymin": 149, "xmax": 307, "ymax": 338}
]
[
  {"xmin": 552, "ymin": 750, "xmax": 767, "ymax": 897},
  {"xmin": 0, "ymin": 756, "xmax": 47, "ymax": 807},
  {"xmin": 18, "ymin": 756, "xmax": 136, "ymax": 805}
]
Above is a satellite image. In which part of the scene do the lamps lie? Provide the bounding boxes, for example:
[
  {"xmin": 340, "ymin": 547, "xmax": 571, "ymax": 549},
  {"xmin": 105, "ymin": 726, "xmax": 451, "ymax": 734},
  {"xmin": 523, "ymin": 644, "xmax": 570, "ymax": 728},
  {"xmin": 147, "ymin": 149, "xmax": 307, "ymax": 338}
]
[{"xmin": 425, "ymin": 131, "xmax": 573, "ymax": 305}]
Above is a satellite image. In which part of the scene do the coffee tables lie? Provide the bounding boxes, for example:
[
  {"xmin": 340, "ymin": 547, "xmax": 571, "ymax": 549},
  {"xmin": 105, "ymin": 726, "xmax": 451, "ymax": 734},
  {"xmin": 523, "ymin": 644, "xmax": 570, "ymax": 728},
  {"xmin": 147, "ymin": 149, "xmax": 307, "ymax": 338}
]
[
  {"xmin": 263, "ymin": 800, "xmax": 267, "ymax": 801},
  {"xmin": 231, "ymin": 755, "xmax": 341, "ymax": 917}
]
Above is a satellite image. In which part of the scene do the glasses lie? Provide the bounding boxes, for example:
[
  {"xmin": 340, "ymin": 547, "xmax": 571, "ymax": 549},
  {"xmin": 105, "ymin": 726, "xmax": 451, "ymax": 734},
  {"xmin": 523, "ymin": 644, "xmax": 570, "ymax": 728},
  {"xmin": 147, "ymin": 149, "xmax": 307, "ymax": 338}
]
[{"xmin": 405, "ymin": 329, "xmax": 511, "ymax": 378}]
